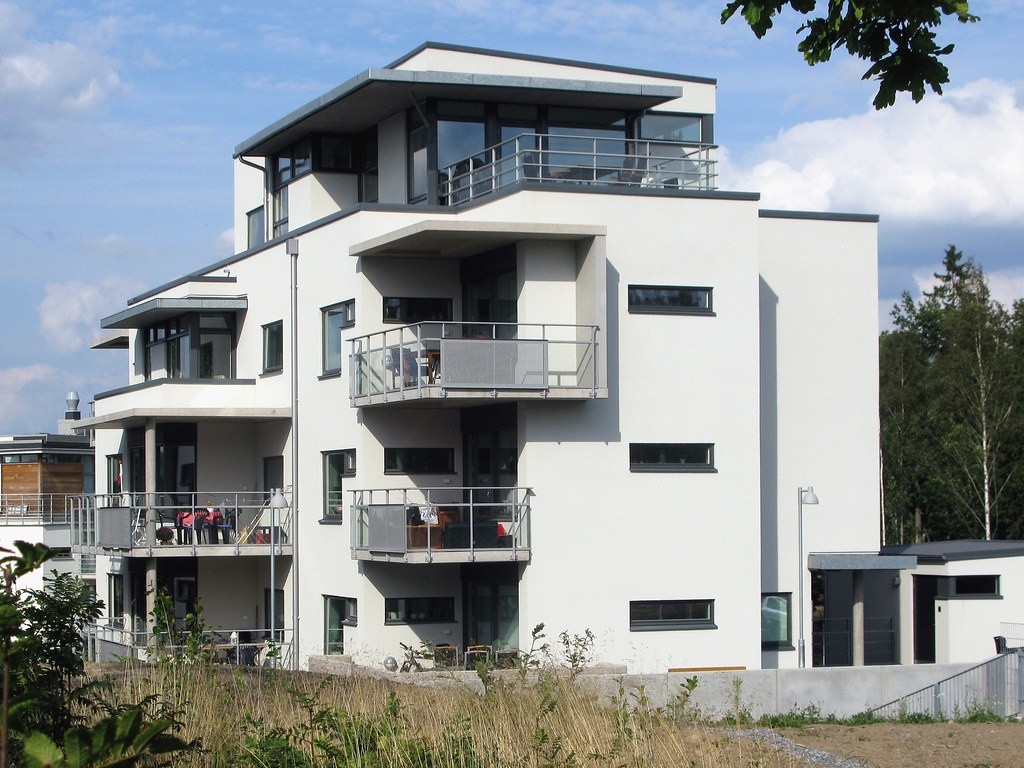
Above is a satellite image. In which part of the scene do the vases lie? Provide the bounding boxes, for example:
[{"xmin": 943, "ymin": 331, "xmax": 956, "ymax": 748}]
[{"xmin": 161, "ymin": 541, "xmax": 172, "ymax": 545}]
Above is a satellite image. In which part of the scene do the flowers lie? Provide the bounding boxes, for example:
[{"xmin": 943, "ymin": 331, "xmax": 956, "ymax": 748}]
[{"xmin": 157, "ymin": 526, "xmax": 174, "ymax": 541}]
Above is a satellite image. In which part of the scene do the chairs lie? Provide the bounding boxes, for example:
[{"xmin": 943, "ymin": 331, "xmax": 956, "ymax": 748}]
[
  {"xmin": 157, "ymin": 507, "xmax": 243, "ymax": 545},
  {"xmin": 435, "ymin": 642, "xmax": 517, "ymax": 670},
  {"xmin": 523, "ymin": 151, "xmax": 539, "ymax": 181},
  {"xmin": 610, "ymin": 151, "xmax": 651, "ymax": 186},
  {"xmin": 390, "ymin": 347, "xmax": 434, "ymax": 389},
  {"xmin": 406, "ymin": 501, "xmax": 498, "ymax": 548}
]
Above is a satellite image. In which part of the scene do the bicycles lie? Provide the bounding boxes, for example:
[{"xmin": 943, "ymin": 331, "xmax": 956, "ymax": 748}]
[{"xmin": 397, "ymin": 641, "xmax": 437, "ymax": 673}]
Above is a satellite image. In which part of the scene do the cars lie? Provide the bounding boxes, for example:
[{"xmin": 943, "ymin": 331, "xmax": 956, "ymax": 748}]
[{"xmin": 762, "ymin": 594, "xmax": 787, "ymax": 635}]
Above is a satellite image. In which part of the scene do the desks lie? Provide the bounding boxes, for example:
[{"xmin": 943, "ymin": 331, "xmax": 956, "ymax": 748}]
[
  {"xmin": 548, "ymin": 165, "xmax": 622, "ymax": 183},
  {"xmin": 403, "ymin": 348, "xmax": 441, "ymax": 386},
  {"xmin": 178, "ymin": 511, "xmax": 226, "ymax": 544}
]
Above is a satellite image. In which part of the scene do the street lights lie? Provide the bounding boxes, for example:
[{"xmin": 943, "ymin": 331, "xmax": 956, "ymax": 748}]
[{"xmin": 798, "ymin": 485, "xmax": 819, "ymax": 668}]
